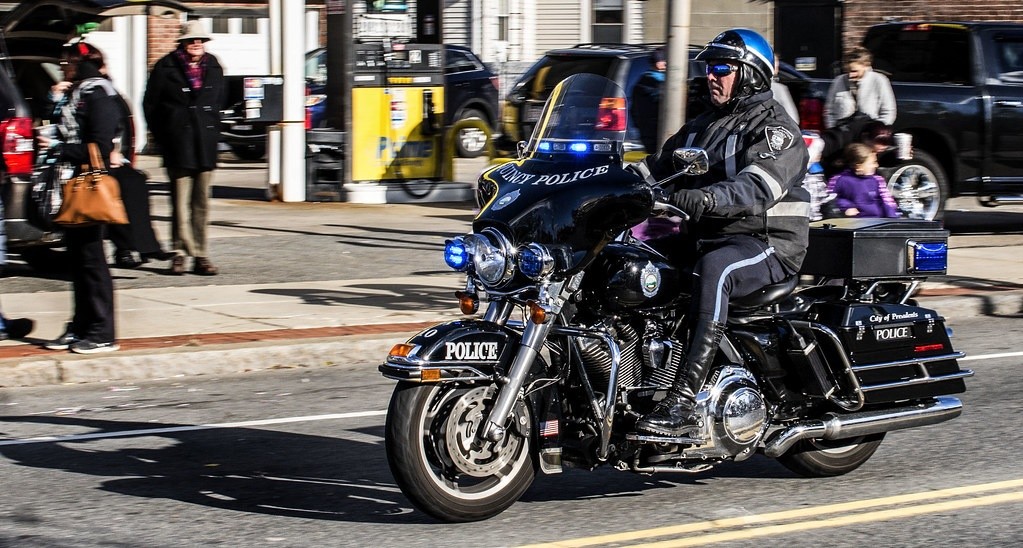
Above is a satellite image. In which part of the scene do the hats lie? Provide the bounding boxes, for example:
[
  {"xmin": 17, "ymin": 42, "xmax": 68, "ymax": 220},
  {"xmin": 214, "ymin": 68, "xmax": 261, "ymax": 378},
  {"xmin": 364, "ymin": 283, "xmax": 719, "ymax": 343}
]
[{"xmin": 176, "ymin": 20, "xmax": 211, "ymax": 43}]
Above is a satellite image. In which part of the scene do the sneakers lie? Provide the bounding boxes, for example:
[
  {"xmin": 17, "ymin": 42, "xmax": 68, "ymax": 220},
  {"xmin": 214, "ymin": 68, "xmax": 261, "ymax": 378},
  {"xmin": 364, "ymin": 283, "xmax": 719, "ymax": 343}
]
[
  {"xmin": 43, "ymin": 334, "xmax": 80, "ymax": 349},
  {"xmin": 70, "ymin": 339, "xmax": 119, "ymax": 353}
]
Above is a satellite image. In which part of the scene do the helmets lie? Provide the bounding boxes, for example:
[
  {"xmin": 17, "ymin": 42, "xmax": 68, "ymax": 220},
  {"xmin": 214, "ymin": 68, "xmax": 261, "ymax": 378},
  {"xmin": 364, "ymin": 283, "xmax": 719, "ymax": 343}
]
[{"xmin": 696, "ymin": 30, "xmax": 775, "ymax": 88}]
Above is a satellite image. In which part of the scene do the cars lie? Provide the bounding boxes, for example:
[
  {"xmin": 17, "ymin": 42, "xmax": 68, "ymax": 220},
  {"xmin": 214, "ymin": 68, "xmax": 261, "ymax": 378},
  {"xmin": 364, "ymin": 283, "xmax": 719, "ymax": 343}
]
[{"xmin": 1, "ymin": 0, "xmax": 198, "ymax": 250}]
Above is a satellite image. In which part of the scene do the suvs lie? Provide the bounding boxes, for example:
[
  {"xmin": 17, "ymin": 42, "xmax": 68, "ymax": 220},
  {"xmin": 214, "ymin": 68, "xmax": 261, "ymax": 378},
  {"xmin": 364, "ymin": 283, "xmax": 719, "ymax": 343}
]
[
  {"xmin": 491, "ymin": 41, "xmax": 840, "ymax": 180},
  {"xmin": 858, "ymin": 18, "xmax": 1023, "ymax": 222},
  {"xmin": 217, "ymin": 34, "xmax": 500, "ymax": 162}
]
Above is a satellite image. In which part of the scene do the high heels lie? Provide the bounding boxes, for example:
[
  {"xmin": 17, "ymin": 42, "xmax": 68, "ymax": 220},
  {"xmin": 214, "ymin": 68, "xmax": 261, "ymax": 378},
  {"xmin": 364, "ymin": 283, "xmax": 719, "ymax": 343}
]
[
  {"xmin": 116, "ymin": 250, "xmax": 142, "ymax": 268},
  {"xmin": 141, "ymin": 249, "xmax": 178, "ymax": 263}
]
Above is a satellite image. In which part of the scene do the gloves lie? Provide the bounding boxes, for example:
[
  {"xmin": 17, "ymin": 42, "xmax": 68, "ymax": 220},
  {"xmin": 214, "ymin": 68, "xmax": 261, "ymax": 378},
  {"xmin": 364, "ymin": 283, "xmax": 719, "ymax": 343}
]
[{"xmin": 668, "ymin": 188, "xmax": 710, "ymax": 222}]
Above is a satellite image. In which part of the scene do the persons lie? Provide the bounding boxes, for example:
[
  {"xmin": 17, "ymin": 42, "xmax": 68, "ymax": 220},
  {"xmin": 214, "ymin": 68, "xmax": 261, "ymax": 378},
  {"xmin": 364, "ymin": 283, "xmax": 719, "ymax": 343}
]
[
  {"xmin": 632, "ymin": 29, "xmax": 810, "ymax": 438},
  {"xmin": 629, "ymin": 45, "xmax": 668, "ymax": 154},
  {"xmin": 0, "ymin": 312, "xmax": 34, "ymax": 340},
  {"xmin": 101, "ymin": 154, "xmax": 179, "ymax": 268},
  {"xmin": 831, "ymin": 142, "xmax": 903, "ymax": 218},
  {"xmin": 37, "ymin": 41, "xmax": 133, "ymax": 354},
  {"xmin": 142, "ymin": 21, "xmax": 226, "ymax": 276},
  {"xmin": 821, "ymin": 48, "xmax": 897, "ymax": 181}
]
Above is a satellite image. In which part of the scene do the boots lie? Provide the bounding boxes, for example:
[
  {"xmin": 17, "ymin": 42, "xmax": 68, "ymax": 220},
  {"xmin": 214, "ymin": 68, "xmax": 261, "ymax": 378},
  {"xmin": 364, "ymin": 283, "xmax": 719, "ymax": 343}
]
[{"xmin": 635, "ymin": 320, "xmax": 726, "ymax": 438}]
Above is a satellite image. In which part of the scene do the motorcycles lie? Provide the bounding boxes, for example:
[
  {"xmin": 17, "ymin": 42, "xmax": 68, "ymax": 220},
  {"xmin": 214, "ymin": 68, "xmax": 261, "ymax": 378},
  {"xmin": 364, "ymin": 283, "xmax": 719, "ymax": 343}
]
[{"xmin": 377, "ymin": 74, "xmax": 975, "ymax": 525}]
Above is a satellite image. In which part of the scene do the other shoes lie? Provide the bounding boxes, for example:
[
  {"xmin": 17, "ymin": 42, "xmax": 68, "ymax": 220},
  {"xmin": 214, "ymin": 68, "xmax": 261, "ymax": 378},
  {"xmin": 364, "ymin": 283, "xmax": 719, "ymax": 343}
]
[
  {"xmin": 194, "ymin": 256, "xmax": 215, "ymax": 274},
  {"xmin": 0, "ymin": 318, "xmax": 33, "ymax": 340},
  {"xmin": 172, "ymin": 256, "xmax": 183, "ymax": 274}
]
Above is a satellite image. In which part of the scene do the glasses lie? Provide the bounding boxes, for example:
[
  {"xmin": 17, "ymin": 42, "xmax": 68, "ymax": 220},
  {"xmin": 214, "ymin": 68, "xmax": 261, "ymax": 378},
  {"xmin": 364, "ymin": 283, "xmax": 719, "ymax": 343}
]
[{"xmin": 705, "ymin": 64, "xmax": 741, "ymax": 77}]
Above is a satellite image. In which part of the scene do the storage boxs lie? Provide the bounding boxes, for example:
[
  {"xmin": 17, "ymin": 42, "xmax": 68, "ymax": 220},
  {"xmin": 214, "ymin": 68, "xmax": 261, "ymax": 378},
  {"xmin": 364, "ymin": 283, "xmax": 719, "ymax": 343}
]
[
  {"xmin": 805, "ymin": 227, "xmax": 949, "ymax": 278},
  {"xmin": 816, "ymin": 302, "xmax": 969, "ymax": 401}
]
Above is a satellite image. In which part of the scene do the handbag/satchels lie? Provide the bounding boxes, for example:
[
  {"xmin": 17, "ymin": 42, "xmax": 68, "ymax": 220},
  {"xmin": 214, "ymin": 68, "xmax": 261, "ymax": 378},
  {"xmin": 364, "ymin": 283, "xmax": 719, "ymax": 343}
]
[
  {"xmin": 54, "ymin": 143, "xmax": 130, "ymax": 225},
  {"xmin": 24, "ymin": 154, "xmax": 76, "ymax": 232}
]
